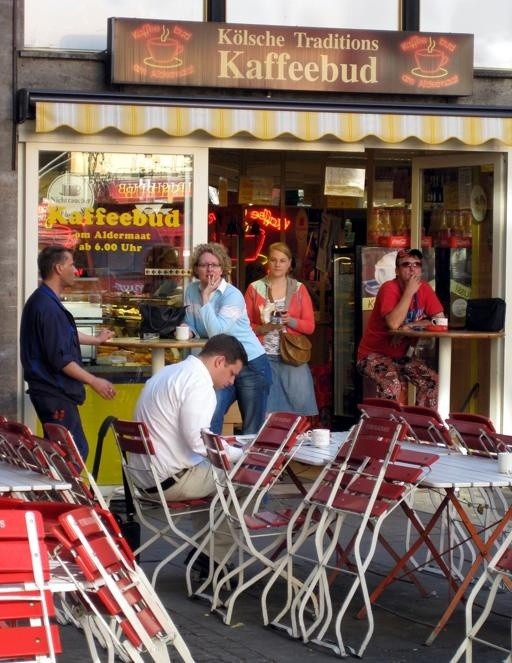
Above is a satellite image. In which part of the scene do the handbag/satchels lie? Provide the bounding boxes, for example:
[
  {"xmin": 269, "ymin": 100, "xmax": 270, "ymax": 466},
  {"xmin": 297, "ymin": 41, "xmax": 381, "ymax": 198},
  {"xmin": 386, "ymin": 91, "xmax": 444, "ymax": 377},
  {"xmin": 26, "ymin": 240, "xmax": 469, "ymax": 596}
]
[
  {"xmin": 465, "ymin": 297, "xmax": 504, "ymax": 333},
  {"xmin": 118, "ymin": 523, "xmax": 140, "ymax": 565},
  {"xmin": 280, "ymin": 333, "xmax": 312, "ymax": 366}
]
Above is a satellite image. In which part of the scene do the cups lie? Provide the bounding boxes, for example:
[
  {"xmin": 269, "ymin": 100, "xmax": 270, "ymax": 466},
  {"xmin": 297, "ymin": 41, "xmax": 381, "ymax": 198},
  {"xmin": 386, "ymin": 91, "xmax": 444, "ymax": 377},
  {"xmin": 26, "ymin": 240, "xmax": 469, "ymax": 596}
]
[
  {"xmin": 498, "ymin": 453, "xmax": 512, "ymax": 474},
  {"xmin": 175, "ymin": 326, "xmax": 190, "ymax": 341},
  {"xmin": 431, "ymin": 318, "xmax": 448, "ymax": 327},
  {"xmin": 306, "ymin": 428, "xmax": 330, "ymax": 447}
]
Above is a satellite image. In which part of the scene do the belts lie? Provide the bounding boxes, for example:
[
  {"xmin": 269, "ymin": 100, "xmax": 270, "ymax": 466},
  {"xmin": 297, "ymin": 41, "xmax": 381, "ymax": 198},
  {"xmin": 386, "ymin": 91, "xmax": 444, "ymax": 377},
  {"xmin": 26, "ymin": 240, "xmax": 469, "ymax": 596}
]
[{"xmin": 142, "ymin": 469, "xmax": 188, "ymax": 492}]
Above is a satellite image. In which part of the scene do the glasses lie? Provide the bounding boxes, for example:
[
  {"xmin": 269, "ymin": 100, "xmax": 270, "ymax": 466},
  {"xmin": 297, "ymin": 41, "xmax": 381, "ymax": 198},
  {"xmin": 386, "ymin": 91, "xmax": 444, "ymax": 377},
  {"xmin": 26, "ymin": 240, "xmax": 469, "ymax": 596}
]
[
  {"xmin": 399, "ymin": 262, "xmax": 422, "ymax": 267},
  {"xmin": 196, "ymin": 262, "xmax": 220, "ymax": 268}
]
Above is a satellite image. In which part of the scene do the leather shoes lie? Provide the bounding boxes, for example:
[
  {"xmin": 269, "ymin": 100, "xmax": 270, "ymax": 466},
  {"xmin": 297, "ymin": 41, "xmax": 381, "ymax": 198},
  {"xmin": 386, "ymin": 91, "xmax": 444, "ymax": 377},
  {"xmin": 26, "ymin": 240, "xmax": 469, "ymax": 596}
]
[{"xmin": 184, "ymin": 549, "xmax": 233, "ymax": 584}]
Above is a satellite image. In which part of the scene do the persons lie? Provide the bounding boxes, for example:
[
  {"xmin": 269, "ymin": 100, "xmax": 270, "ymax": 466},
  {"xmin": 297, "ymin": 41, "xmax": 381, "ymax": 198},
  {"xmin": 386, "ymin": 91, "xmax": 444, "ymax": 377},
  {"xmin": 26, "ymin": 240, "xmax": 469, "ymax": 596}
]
[
  {"xmin": 17, "ymin": 244, "xmax": 118, "ymax": 474},
  {"xmin": 122, "ymin": 331, "xmax": 265, "ymax": 594},
  {"xmin": 180, "ymin": 241, "xmax": 273, "ymax": 436},
  {"xmin": 244, "ymin": 239, "xmax": 321, "ymax": 422},
  {"xmin": 138, "ymin": 242, "xmax": 186, "ymax": 337},
  {"xmin": 354, "ymin": 246, "xmax": 448, "ymax": 418}
]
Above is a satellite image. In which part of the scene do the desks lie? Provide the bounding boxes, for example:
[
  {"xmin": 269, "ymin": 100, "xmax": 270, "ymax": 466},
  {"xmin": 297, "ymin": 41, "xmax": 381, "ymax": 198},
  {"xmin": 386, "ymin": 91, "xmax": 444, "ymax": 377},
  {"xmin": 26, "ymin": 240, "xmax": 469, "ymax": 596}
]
[
  {"xmin": 103, "ymin": 336, "xmax": 208, "ymax": 377},
  {"xmin": 388, "ymin": 328, "xmax": 505, "ymax": 429}
]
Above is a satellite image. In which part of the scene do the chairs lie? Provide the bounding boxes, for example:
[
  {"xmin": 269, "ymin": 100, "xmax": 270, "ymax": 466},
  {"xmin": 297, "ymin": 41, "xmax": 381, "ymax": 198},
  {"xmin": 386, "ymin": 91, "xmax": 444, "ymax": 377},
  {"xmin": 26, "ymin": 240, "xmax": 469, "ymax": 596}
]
[{"xmin": 0, "ymin": 397, "xmax": 512, "ymax": 662}]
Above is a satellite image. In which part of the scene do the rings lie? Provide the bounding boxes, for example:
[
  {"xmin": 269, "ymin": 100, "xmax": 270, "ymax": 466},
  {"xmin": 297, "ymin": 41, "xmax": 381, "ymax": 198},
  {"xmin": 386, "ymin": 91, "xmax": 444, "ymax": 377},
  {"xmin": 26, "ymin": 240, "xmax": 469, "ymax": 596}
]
[
  {"xmin": 210, "ymin": 281, "xmax": 215, "ymax": 287},
  {"xmin": 108, "ymin": 388, "xmax": 113, "ymax": 392}
]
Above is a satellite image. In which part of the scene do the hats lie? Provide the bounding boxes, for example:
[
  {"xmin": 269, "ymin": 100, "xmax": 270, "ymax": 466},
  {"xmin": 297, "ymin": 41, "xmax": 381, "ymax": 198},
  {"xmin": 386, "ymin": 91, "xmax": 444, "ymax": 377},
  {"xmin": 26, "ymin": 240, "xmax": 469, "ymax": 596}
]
[{"xmin": 396, "ymin": 248, "xmax": 424, "ymax": 260}]
